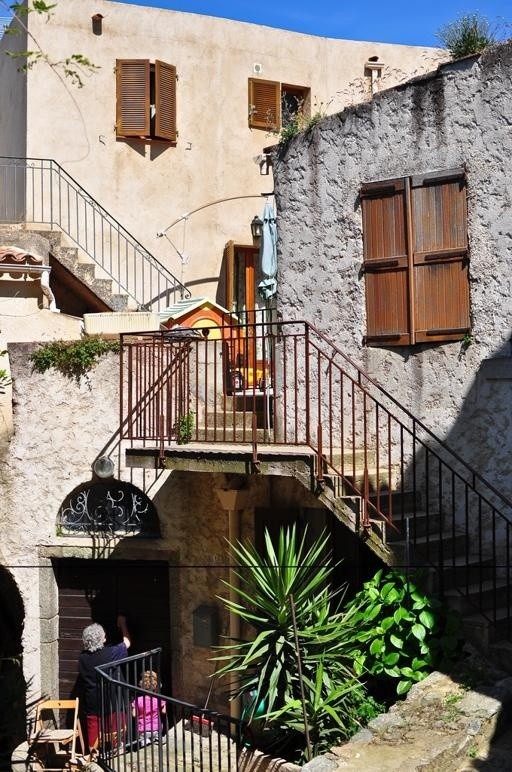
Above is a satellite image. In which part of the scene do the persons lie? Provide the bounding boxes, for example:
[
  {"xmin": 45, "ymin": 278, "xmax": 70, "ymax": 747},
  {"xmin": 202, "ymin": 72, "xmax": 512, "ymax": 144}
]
[
  {"xmin": 130, "ymin": 670, "xmax": 166, "ymax": 747},
  {"xmin": 77, "ymin": 615, "xmax": 132, "ymax": 754}
]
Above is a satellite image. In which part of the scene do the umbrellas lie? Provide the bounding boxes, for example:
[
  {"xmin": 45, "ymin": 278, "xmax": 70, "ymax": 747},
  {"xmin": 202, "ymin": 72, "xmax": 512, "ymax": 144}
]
[{"xmin": 257, "ymin": 195, "xmax": 278, "ymax": 438}]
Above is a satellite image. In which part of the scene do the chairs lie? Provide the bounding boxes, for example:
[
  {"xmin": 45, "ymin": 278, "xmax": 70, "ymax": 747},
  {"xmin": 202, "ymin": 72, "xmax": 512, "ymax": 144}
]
[{"xmin": 27, "ymin": 696, "xmax": 87, "ymax": 772}]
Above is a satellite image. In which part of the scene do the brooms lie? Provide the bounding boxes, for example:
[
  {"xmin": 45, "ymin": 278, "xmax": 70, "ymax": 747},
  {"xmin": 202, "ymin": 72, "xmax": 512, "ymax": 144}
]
[{"xmin": 183, "ymin": 630, "xmax": 228, "ymax": 737}]
